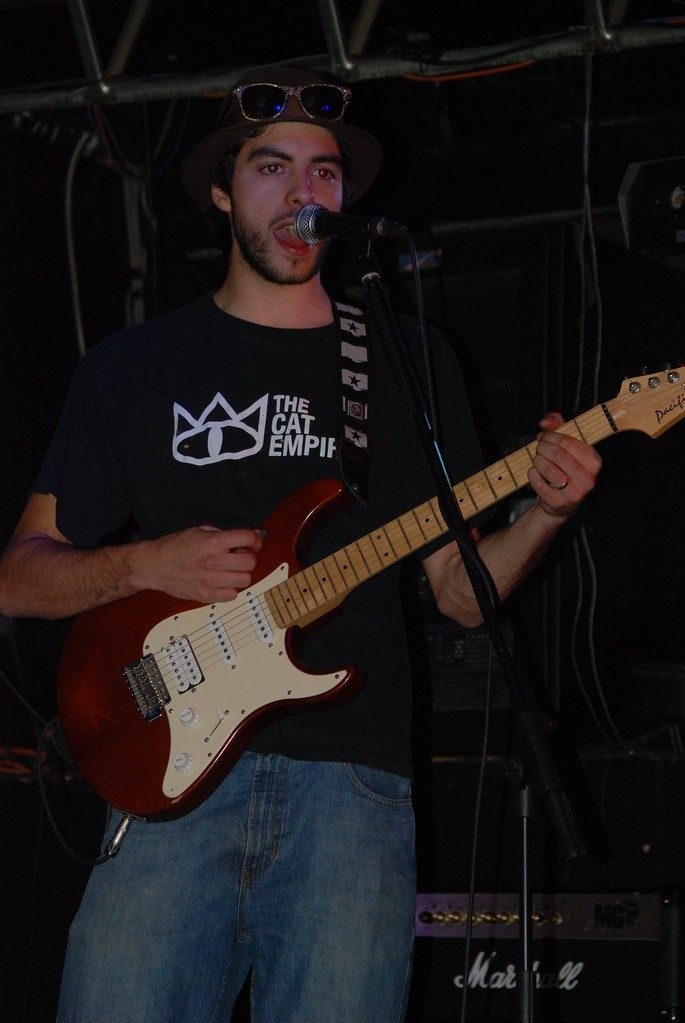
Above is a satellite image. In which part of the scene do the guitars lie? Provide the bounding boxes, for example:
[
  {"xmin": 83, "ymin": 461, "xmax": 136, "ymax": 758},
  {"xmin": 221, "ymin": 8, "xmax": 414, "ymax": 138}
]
[{"xmin": 54, "ymin": 364, "xmax": 685, "ymax": 823}]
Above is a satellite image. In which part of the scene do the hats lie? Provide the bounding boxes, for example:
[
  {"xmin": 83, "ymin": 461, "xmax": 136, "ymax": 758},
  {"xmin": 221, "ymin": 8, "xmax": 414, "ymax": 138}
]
[{"xmin": 178, "ymin": 67, "xmax": 384, "ymax": 213}]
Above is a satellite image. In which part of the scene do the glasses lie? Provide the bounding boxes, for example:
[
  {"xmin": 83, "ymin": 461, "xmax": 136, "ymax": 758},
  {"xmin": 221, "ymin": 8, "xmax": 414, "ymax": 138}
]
[{"xmin": 215, "ymin": 83, "xmax": 353, "ymax": 122}]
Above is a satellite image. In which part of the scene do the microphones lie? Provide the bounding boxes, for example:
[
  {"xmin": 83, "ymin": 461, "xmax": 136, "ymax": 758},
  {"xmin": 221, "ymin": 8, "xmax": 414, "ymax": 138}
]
[{"xmin": 294, "ymin": 202, "xmax": 404, "ymax": 245}]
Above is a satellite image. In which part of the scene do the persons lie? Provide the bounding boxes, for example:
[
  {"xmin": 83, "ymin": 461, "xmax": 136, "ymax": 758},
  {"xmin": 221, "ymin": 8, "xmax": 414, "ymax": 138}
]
[{"xmin": 0, "ymin": 66, "xmax": 603, "ymax": 1023}]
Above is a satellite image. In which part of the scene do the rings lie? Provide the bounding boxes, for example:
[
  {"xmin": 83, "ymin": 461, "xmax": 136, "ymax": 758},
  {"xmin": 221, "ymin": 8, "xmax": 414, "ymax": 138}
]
[{"xmin": 558, "ymin": 477, "xmax": 568, "ymax": 489}]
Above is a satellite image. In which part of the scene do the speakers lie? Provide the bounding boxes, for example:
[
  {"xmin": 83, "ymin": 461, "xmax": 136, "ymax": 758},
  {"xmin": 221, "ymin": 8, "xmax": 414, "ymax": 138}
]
[{"xmin": 403, "ymin": 882, "xmax": 684, "ymax": 1022}]
